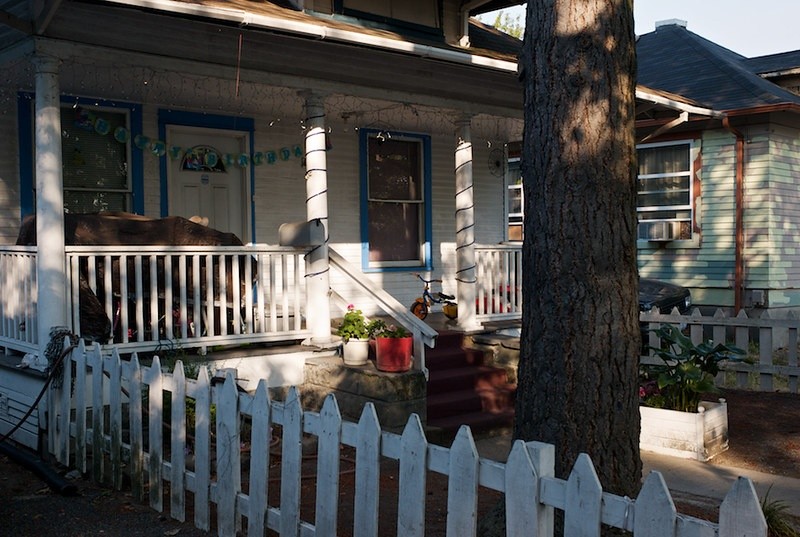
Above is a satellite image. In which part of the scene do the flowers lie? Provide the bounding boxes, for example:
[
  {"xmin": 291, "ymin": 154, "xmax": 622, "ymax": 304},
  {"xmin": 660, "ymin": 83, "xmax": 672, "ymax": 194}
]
[
  {"xmin": 368, "ymin": 320, "xmax": 407, "ymax": 339},
  {"xmin": 337, "ymin": 304, "xmax": 371, "ymax": 340}
]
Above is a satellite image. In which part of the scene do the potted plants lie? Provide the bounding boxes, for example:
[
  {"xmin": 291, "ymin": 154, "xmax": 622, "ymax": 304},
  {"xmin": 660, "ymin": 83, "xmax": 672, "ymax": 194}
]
[{"xmin": 635, "ymin": 321, "xmax": 754, "ymax": 461}]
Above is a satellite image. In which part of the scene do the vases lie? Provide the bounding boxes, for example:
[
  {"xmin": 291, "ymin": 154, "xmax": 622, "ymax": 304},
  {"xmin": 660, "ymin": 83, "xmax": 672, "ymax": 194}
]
[
  {"xmin": 341, "ymin": 337, "xmax": 370, "ymax": 366},
  {"xmin": 377, "ymin": 336, "xmax": 412, "ymax": 373}
]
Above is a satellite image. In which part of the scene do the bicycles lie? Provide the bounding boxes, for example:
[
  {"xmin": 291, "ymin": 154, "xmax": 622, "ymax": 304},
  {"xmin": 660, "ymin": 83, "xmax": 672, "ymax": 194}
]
[{"xmin": 410, "ymin": 273, "xmax": 458, "ymax": 321}]
